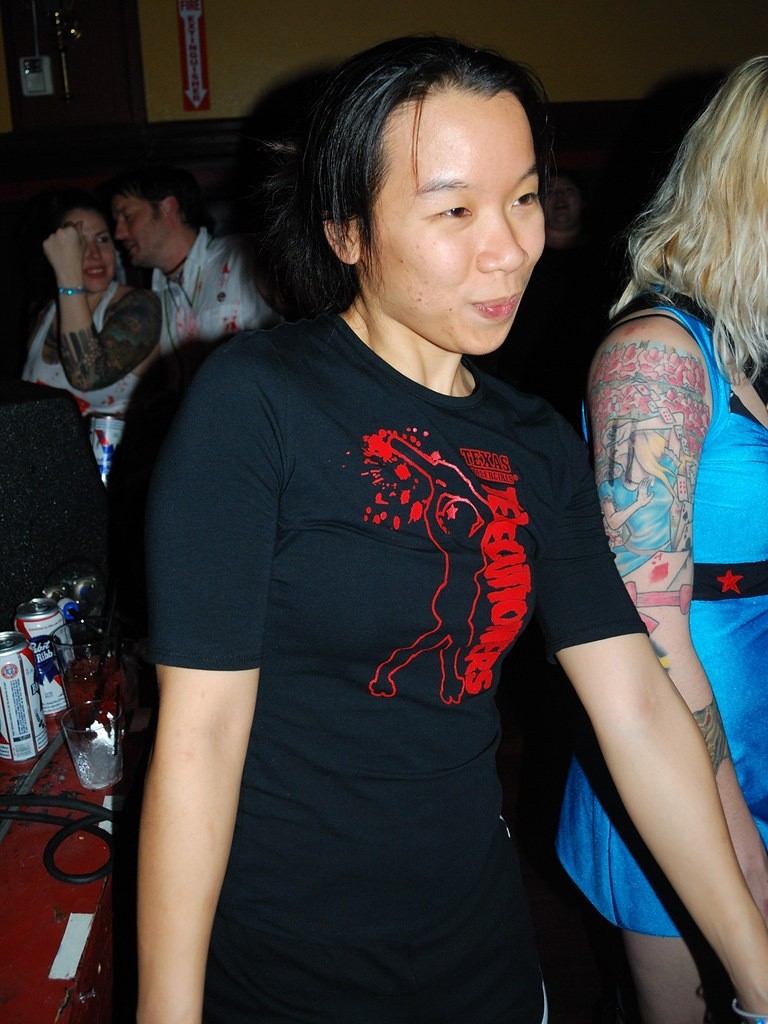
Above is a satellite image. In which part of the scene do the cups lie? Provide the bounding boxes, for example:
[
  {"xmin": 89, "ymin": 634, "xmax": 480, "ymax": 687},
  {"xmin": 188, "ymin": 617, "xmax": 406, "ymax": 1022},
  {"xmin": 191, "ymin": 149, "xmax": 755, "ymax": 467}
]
[
  {"xmin": 61, "ymin": 699, "xmax": 125, "ymax": 790},
  {"xmin": 50, "ymin": 620, "xmax": 128, "ymax": 721}
]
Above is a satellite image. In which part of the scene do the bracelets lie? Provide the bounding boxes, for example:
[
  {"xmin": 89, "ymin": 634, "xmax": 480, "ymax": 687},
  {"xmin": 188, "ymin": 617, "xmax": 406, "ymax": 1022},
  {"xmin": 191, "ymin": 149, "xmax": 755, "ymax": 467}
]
[
  {"xmin": 57, "ymin": 286, "xmax": 87, "ymax": 297},
  {"xmin": 730, "ymin": 997, "xmax": 768, "ymax": 1024}
]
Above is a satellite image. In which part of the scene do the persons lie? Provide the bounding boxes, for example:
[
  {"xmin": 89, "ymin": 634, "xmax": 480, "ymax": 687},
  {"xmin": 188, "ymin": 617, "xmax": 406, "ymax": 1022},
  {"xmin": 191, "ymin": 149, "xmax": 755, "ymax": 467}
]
[
  {"xmin": 132, "ymin": 30, "xmax": 767, "ymax": 1024},
  {"xmin": 503, "ymin": 168, "xmax": 630, "ymax": 426},
  {"xmin": 1, "ymin": 169, "xmax": 282, "ymax": 494},
  {"xmin": 548, "ymin": 57, "xmax": 768, "ymax": 1024}
]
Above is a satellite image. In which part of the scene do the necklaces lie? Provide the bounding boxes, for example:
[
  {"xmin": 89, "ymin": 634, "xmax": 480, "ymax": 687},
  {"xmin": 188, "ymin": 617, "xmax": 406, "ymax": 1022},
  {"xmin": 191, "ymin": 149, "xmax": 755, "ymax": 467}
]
[{"xmin": 163, "ymin": 255, "xmax": 187, "ymax": 285}]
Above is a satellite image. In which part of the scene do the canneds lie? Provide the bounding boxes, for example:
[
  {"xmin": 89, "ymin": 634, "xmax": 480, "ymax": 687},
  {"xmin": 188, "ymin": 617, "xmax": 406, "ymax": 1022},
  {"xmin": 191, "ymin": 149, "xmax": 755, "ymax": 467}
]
[
  {"xmin": 0, "ymin": 630, "xmax": 50, "ymax": 766},
  {"xmin": 88, "ymin": 412, "xmax": 127, "ymax": 485},
  {"xmin": 14, "ymin": 597, "xmax": 78, "ymax": 718}
]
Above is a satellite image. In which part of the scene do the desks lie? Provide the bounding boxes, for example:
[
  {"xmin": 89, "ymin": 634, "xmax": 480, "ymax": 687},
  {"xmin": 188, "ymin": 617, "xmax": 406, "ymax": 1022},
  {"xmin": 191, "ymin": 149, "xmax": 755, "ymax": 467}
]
[{"xmin": 0, "ymin": 635, "xmax": 121, "ymax": 1024}]
